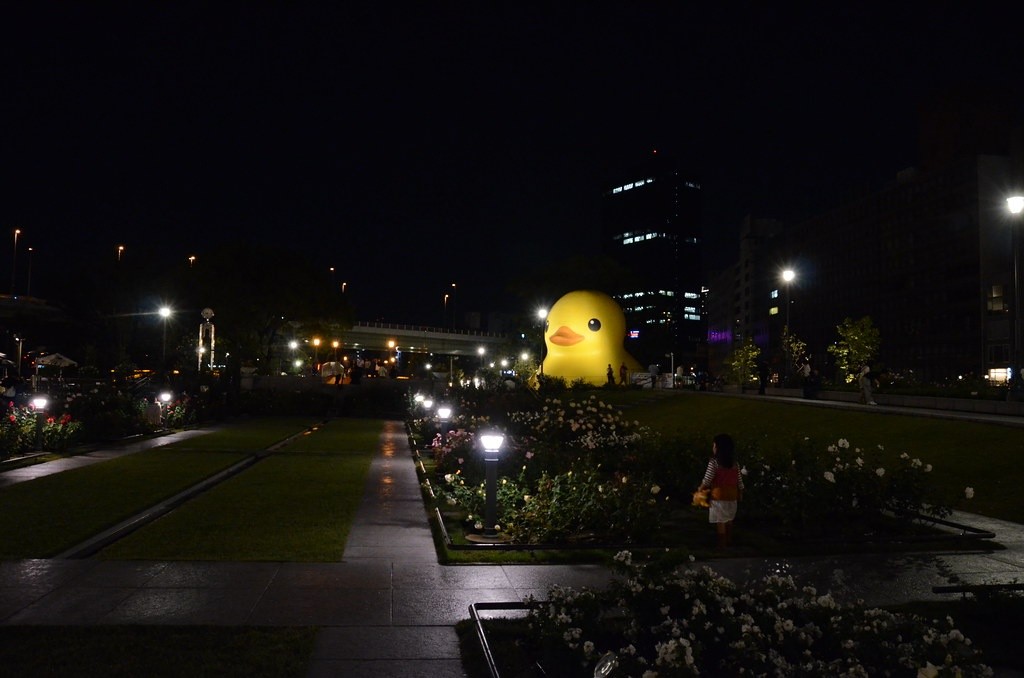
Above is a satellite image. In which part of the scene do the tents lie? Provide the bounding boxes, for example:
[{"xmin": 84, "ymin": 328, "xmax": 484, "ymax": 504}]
[
  {"xmin": 0, "ymin": 353, "xmax": 8, "ymax": 378},
  {"xmin": 35, "ymin": 353, "xmax": 77, "ymax": 381}
]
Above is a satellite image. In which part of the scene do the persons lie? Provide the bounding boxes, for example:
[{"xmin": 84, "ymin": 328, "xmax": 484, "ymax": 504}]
[
  {"xmin": 797, "ymin": 360, "xmax": 811, "ymax": 396},
  {"xmin": 751, "ymin": 359, "xmax": 771, "ymax": 396},
  {"xmin": 618, "ymin": 362, "xmax": 628, "ymax": 388},
  {"xmin": 607, "ymin": 364, "xmax": 614, "ymax": 384},
  {"xmin": 334, "ymin": 361, "xmax": 345, "ymax": 385},
  {"xmin": 675, "ymin": 365, "xmax": 685, "ymax": 389},
  {"xmin": 859, "ymin": 373, "xmax": 869, "ymax": 402},
  {"xmin": 696, "ymin": 372, "xmax": 723, "ymax": 393},
  {"xmin": 311, "ymin": 365, "xmax": 318, "ymax": 377},
  {"xmin": 349, "ymin": 347, "xmax": 407, "ymax": 379},
  {"xmin": 698, "ymin": 433, "xmax": 744, "ymax": 551},
  {"xmin": 650, "ymin": 364, "xmax": 659, "ymax": 389}
]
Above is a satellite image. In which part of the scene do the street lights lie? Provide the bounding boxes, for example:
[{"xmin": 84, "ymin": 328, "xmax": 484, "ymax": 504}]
[
  {"xmin": 444, "ymin": 294, "xmax": 449, "ymax": 333},
  {"xmin": 154, "ymin": 303, "xmax": 175, "ymax": 360},
  {"xmin": 665, "ymin": 352, "xmax": 675, "ymax": 390},
  {"xmin": 534, "ymin": 304, "xmax": 550, "ymax": 375},
  {"xmin": 12, "ymin": 229, "xmax": 21, "ymax": 294},
  {"xmin": 386, "ymin": 339, "xmax": 396, "ymax": 362},
  {"xmin": 1000, "ymin": 190, "xmax": 1023, "ymax": 403},
  {"xmin": 26, "ymin": 247, "xmax": 33, "ymax": 297},
  {"xmin": 287, "ymin": 339, "xmax": 301, "ymax": 360},
  {"xmin": 311, "ymin": 338, "xmax": 321, "ymax": 363},
  {"xmin": 117, "ymin": 245, "xmax": 124, "ymax": 313},
  {"xmin": 451, "ymin": 283, "xmax": 457, "ymax": 332},
  {"xmin": 449, "ymin": 356, "xmax": 459, "ymax": 382},
  {"xmin": 777, "ymin": 265, "xmax": 801, "ymax": 388},
  {"xmin": 331, "ymin": 340, "xmax": 339, "ymax": 362},
  {"xmin": 187, "ymin": 255, "xmax": 196, "ymax": 309},
  {"xmin": 475, "ymin": 346, "xmax": 488, "ymax": 367},
  {"xmin": 425, "ymin": 363, "xmax": 432, "ymax": 378}
]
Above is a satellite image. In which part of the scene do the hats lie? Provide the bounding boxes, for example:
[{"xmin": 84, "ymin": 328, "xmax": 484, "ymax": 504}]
[{"xmin": 649, "ymin": 360, "xmax": 655, "ymax": 364}]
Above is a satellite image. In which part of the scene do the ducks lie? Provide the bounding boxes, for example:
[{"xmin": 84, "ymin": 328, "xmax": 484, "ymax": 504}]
[{"xmin": 539, "ymin": 292, "xmax": 642, "ymax": 385}]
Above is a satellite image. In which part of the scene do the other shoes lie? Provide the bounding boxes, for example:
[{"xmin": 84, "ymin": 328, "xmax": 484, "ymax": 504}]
[{"xmin": 713, "ymin": 545, "xmax": 729, "ymax": 552}]
